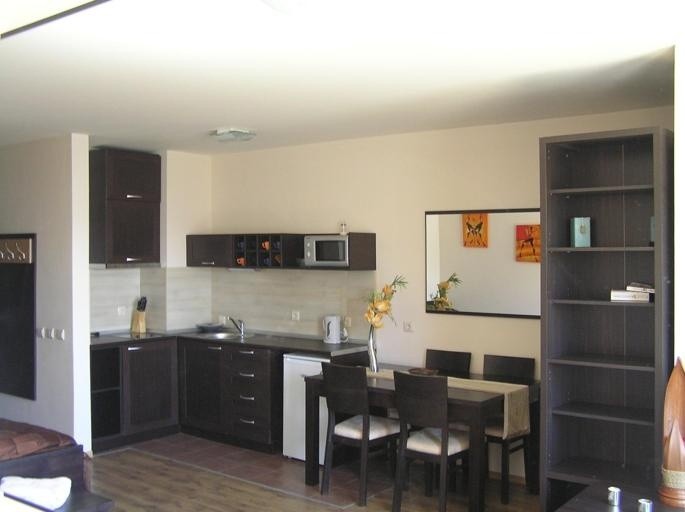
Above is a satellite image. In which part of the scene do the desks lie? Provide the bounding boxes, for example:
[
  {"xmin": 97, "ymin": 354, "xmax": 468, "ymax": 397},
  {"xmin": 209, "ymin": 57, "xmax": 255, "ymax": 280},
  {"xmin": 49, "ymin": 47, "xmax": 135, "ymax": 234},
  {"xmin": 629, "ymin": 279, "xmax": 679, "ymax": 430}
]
[{"xmin": 553, "ymin": 481, "xmax": 662, "ymax": 512}]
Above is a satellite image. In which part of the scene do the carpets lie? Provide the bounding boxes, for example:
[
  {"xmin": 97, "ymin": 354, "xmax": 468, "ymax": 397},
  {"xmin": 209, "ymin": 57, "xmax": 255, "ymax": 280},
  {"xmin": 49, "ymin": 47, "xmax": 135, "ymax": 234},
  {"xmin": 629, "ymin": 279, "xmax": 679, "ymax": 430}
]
[{"xmin": 94, "ymin": 447, "xmax": 350, "ymax": 511}]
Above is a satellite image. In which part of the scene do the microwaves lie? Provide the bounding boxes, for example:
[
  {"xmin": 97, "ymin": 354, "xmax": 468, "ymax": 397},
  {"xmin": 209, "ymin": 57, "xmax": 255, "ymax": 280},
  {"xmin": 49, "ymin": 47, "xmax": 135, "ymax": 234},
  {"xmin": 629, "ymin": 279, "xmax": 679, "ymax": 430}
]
[{"xmin": 303, "ymin": 235, "xmax": 349, "ymax": 268}]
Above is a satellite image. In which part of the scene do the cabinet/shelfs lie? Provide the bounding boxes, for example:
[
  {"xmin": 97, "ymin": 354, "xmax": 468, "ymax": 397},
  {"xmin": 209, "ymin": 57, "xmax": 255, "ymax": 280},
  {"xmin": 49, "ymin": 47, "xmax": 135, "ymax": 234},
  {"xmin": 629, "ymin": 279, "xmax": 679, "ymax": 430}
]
[
  {"xmin": 177, "ymin": 339, "xmax": 282, "ymax": 457},
  {"xmin": 91, "ymin": 329, "xmax": 179, "ymax": 451},
  {"xmin": 186, "ymin": 232, "xmax": 376, "ymax": 272},
  {"xmin": 88, "ymin": 146, "xmax": 161, "ymax": 268},
  {"xmin": 538, "ymin": 125, "xmax": 674, "ymax": 511}
]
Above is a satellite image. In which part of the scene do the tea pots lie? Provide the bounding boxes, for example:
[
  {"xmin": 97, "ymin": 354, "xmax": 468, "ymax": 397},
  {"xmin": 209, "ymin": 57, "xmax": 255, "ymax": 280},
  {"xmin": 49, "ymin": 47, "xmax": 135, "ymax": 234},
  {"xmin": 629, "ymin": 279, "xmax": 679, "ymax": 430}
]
[{"xmin": 321, "ymin": 315, "xmax": 341, "ymax": 344}]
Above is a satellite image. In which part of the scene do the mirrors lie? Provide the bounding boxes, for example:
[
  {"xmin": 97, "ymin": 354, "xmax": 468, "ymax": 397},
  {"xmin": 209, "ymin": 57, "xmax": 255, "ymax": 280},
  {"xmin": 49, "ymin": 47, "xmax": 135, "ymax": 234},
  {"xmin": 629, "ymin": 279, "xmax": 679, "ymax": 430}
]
[{"xmin": 425, "ymin": 207, "xmax": 541, "ymax": 319}]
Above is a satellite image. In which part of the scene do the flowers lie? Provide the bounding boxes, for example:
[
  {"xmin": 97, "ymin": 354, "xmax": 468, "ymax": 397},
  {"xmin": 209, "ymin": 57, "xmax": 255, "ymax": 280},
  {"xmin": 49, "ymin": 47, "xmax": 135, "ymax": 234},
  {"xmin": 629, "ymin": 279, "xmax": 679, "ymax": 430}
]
[
  {"xmin": 363, "ymin": 272, "xmax": 407, "ymax": 329},
  {"xmin": 433, "ymin": 274, "xmax": 463, "ymax": 312}
]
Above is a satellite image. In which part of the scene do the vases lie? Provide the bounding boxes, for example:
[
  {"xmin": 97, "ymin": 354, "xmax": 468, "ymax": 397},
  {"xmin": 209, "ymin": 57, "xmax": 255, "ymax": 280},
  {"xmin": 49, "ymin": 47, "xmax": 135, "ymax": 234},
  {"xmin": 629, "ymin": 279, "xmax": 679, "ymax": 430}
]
[{"xmin": 369, "ymin": 326, "xmax": 380, "ymax": 372}]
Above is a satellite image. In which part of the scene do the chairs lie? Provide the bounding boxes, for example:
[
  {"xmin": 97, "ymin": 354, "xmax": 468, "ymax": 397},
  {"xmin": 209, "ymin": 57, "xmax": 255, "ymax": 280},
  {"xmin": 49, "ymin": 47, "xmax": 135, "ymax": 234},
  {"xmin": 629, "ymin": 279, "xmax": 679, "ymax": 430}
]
[
  {"xmin": 392, "ymin": 370, "xmax": 490, "ymax": 512},
  {"xmin": 387, "ymin": 349, "xmax": 473, "ymax": 483},
  {"xmin": 448, "ymin": 354, "xmax": 536, "ymax": 505},
  {"xmin": 320, "ymin": 363, "xmax": 413, "ymax": 509}
]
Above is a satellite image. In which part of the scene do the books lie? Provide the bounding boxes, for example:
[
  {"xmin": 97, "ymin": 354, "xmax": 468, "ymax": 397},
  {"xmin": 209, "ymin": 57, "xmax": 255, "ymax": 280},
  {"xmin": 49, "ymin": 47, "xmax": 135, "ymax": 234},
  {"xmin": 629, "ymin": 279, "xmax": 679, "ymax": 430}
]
[
  {"xmin": 611, "ymin": 290, "xmax": 649, "ymax": 303},
  {"xmin": 626, "ymin": 282, "xmax": 654, "ymax": 293}
]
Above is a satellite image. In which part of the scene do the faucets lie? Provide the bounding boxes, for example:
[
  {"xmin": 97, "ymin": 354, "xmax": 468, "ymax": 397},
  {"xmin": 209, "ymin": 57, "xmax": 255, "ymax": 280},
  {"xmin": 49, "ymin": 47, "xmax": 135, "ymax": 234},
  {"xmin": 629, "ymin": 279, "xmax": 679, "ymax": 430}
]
[{"xmin": 226, "ymin": 314, "xmax": 246, "ymax": 335}]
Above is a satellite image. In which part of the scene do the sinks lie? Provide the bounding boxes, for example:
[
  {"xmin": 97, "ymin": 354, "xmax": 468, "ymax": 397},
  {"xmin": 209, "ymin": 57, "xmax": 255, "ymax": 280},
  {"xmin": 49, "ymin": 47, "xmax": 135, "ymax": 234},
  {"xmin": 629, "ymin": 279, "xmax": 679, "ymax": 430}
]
[{"xmin": 199, "ymin": 331, "xmax": 255, "ymax": 340}]
